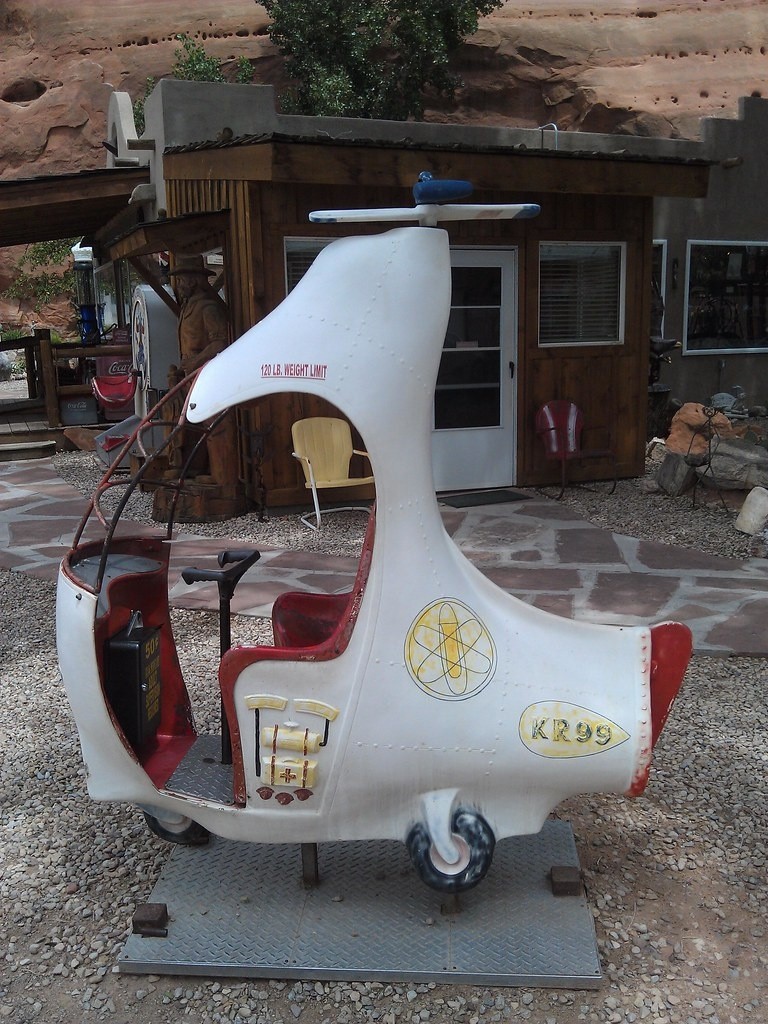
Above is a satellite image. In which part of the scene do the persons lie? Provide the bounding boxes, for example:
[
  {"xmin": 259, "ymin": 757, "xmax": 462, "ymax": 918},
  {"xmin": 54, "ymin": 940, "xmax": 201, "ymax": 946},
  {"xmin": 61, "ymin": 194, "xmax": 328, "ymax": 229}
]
[{"xmin": 164, "ymin": 254, "xmax": 238, "ymax": 485}]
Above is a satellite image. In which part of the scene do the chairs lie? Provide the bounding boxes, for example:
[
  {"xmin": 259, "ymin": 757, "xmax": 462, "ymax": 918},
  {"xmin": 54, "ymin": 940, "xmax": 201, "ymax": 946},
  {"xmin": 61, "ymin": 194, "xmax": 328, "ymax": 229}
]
[
  {"xmin": 535, "ymin": 400, "xmax": 618, "ymax": 500},
  {"xmin": 291, "ymin": 417, "xmax": 376, "ymax": 532}
]
